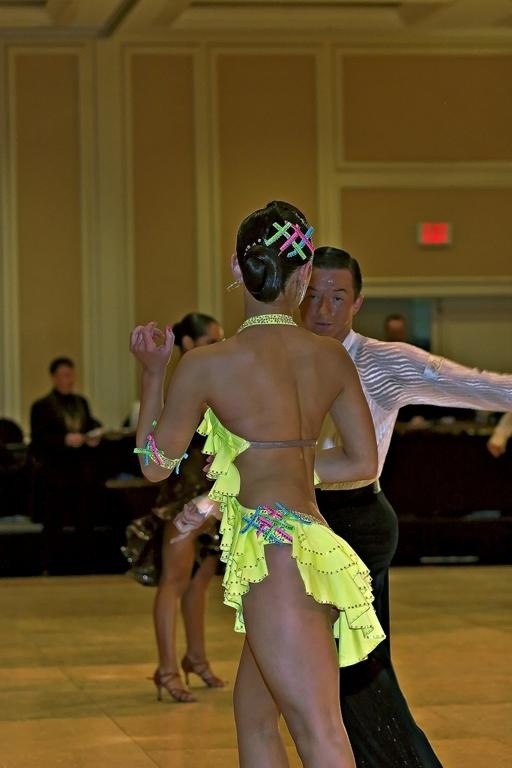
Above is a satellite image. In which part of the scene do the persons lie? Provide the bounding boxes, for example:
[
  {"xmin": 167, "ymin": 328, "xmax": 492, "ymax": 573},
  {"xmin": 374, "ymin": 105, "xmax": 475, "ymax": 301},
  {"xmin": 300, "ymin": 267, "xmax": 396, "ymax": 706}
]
[
  {"xmin": 382, "ymin": 309, "xmax": 441, "ymax": 426},
  {"xmin": 126, "ymin": 200, "xmax": 382, "ymax": 768},
  {"xmin": 485, "ymin": 412, "xmax": 510, "ymax": 460},
  {"xmin": 28, "ymin": 356, "xmax": 108, "ymax": 580},
  {"xmin": 201, "ymin": 246, "xmax": 509, "ymax": 768},
  {"xmin": 152, "ymin": 306, "xmax": 230, "ymax": 701}
]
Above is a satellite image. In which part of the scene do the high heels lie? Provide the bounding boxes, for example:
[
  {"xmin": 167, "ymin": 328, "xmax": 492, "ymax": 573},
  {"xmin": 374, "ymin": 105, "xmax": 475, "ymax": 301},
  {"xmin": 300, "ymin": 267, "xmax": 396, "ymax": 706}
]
[
  {"xmin": 181, "ymin": 651, "xmax": 228, "ymax": 687},
  {"xmin": 154, "ymin": 667, "xmax": 197, "ymax": 704}
]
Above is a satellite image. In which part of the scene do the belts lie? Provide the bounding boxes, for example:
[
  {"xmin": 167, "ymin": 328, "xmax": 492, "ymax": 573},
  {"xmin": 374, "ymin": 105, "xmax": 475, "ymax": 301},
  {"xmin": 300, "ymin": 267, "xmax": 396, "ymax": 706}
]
[{"xmin": 316, "ymin": 481, "xmax": 381, "ymax": 499}]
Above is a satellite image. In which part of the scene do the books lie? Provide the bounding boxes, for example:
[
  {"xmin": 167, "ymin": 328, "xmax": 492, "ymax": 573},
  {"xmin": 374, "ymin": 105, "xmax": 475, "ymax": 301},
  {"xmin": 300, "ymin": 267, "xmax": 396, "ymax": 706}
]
[{"xmin": 85, "ymin": 424, "xmax": 110, "ymax": 442}]
